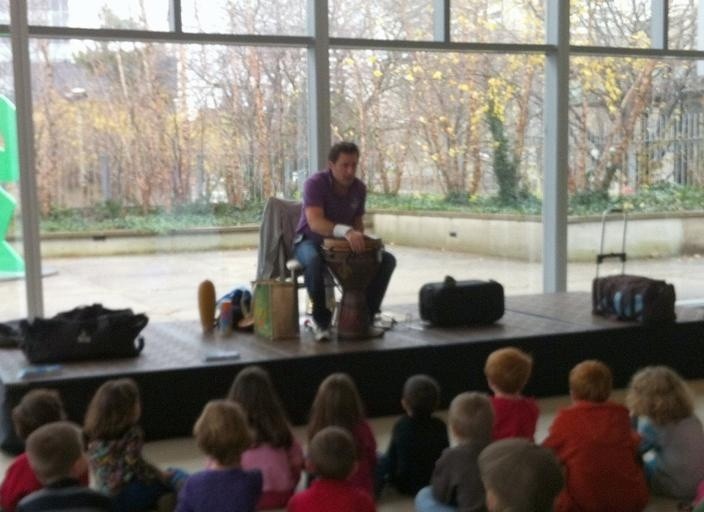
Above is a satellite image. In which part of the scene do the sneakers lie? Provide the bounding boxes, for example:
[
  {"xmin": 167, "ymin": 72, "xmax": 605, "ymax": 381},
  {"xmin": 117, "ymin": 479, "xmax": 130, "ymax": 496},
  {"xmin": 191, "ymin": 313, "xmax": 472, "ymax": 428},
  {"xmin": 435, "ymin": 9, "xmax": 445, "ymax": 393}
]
[
  {"xmin": 311, "ymin": 321, "xmax": 331, "ymax": 342},
  {"xmin": 337, "ymin": 326, "xmax": 384, "ymax": 340}
]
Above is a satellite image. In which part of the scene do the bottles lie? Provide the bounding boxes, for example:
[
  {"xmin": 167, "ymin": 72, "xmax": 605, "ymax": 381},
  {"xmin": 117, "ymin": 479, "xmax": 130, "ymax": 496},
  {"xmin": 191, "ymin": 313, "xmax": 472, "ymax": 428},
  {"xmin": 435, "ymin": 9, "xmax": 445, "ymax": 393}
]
[{"xmin": 217, "ymin": 294, "xmax": 232, "ymax": 339}]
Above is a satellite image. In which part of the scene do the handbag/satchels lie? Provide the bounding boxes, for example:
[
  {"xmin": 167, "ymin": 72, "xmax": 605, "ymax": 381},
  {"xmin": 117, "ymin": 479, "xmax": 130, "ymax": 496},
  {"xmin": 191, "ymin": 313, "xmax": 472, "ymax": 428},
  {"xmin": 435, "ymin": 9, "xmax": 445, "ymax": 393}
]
[
  {"xmin": 18, "ymin": 304, "xmax": 148, "ymax": 365},
  {"xmin": 594, "ymin": 274, "xmax": 676, "ymax": 325},
  {"xmin": 214, "ymin": 289, "xmax": 252, "ymax": 332}
]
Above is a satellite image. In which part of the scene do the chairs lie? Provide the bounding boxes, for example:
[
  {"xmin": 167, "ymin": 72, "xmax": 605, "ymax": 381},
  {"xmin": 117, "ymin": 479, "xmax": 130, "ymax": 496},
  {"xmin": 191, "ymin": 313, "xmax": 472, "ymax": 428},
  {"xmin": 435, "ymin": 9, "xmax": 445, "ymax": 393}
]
[{"xmin": 267, "ymin": 196, "xmax": 341, "ymax": 339}]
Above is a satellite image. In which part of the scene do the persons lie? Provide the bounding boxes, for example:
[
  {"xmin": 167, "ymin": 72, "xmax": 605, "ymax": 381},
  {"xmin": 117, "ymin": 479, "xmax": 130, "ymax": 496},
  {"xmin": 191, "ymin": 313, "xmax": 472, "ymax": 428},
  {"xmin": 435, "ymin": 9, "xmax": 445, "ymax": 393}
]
[
  {"xmin": 538, "ymin": 358, "xmax": 650, "ymax": 512},
  {"xmin": 304, "ymin": 372, "xmax": 389, "ymax": 502},
  {"xmin": 476, "ymin": 435, "xmax": 568, "ymax": 511},
  {"xmin": 284, "ymin": 427, "xmax": 375, "ymax": 511},
  {"xmin": 485, "ymin": 347, "xmax": 541, "ymax": 444},
  {"xmin": 83, "ymin": 378, "xmax": 179, "ymax": 512},
  {"xmin": 0, "ymin": 389, "xmax": 90, "ymax": 511},
  {"xmin": 290, "ymin": 141, "xmax": 396, "ymax": 341},
  {"xmin": 205, "ymin": 366, "xmax": 304, "ymax": 509},
  {"xmin": 384, "ymin": 374, "xmax": 449, "ymax": 495},
  {"xmin": 414, "ymin": 392, "xmax": 495, "ymax": 512},
  {"xmin": 626, "ymin": 366, "xmax": 703, "ymax": 500},
  {"xmin": 173, "ymin": 398, "xmax": 264, "ymax": 512},
  {"xmin": 14, "ymin": 422, "xmax": 119, "ymax": 510}
]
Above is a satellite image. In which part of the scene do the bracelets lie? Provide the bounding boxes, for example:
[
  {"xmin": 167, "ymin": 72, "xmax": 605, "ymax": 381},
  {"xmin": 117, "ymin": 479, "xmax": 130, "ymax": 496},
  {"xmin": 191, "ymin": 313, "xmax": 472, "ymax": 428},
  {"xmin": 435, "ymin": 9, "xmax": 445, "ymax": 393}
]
[{"xmin": 332, "ymin": 223, "xmax": 352, "ymax": 239}]
